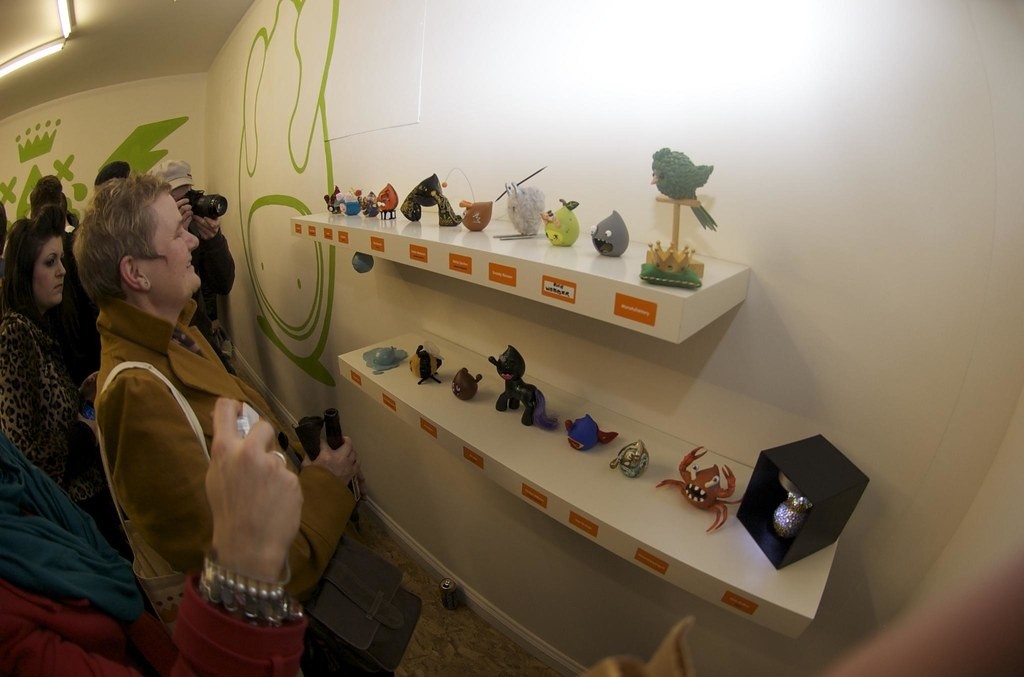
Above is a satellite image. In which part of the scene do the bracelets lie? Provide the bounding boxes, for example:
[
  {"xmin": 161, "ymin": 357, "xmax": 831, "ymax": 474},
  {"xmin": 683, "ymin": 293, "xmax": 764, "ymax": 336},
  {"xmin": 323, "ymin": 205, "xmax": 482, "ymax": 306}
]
[{"xmin": 200, "ymin": 556, "xmax": 303, "ymax": 625}]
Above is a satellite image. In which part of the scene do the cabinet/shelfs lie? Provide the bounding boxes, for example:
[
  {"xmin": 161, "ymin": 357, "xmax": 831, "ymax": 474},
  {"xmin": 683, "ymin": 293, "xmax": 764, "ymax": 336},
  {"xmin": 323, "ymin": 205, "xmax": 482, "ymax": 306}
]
[{"xmin": 289, "ymin": 215, "xmax": 840, "ymax": 636}]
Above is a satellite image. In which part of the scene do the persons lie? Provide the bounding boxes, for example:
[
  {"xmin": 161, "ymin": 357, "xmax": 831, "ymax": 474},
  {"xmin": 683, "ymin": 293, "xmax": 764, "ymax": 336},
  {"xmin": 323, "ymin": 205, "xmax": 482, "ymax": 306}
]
[
  {"xmin": 1, "ymin": 159, "xmax": 363, "ymax": 675},
  {"xmin": 72, "ymin": 175, "xmax": 359, "ymax": 636}
]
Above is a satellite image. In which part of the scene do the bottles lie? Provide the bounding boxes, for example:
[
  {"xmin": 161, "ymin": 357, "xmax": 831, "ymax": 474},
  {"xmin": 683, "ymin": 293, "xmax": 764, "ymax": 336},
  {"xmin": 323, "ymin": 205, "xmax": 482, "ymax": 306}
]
[{"xmin": 324, "ymin": 408, "xmax": 362, "ymax": 503}]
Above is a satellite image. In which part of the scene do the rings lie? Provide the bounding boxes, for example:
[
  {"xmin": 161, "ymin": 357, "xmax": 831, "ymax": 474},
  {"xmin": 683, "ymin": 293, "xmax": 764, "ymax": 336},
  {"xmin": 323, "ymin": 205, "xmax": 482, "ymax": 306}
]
[{"xmin": 270, "ymin": 449, "xmax": 288, "ymax": 466}]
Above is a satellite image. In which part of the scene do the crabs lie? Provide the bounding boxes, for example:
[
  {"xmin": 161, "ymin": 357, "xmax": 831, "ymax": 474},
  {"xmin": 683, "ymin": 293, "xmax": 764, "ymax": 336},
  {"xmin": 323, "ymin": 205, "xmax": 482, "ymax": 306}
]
[{"xmin": 655, "ymin": 440, "xmax": 749, "ymax": 534}]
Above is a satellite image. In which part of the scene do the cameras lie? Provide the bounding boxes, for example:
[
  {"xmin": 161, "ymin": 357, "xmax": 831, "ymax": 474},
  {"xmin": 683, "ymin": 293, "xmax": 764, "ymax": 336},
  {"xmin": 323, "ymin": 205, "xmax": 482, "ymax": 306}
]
[{"xmin": 179, "ymin": 190, "xmax": 228, "ymax": 220}]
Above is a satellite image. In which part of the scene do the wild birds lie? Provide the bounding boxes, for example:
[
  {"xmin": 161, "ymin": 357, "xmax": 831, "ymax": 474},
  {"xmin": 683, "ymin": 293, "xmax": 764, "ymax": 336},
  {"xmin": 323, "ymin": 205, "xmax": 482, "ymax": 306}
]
[{"xmin": 650, "ymin": 145, "xmax": 723, "ymax": 229}]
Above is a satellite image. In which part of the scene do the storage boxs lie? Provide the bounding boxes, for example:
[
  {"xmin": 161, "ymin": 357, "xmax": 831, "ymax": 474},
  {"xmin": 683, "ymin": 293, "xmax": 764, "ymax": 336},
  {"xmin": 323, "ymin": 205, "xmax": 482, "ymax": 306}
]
[{"xmin": 735, "ymin": 434, "xmax": 869, "ymax": 570}]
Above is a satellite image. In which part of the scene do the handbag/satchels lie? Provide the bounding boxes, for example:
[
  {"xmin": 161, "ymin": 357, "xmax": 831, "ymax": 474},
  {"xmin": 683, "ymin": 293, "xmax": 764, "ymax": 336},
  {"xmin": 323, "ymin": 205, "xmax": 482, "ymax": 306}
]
[
  {"xmin": 94, "ymin": 360, "xmax": 212, "ymax": 626},
  {"xmin": 297, "ymin": 536, "xmax": 423, "ymax": 677}
]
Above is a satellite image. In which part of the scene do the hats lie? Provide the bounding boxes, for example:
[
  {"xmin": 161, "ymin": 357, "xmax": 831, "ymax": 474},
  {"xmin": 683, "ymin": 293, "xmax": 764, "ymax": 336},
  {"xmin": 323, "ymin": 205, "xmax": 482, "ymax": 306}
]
[{"xmin": 151, "ymin": 160, "xmax": 194, "ymax": 191}]
